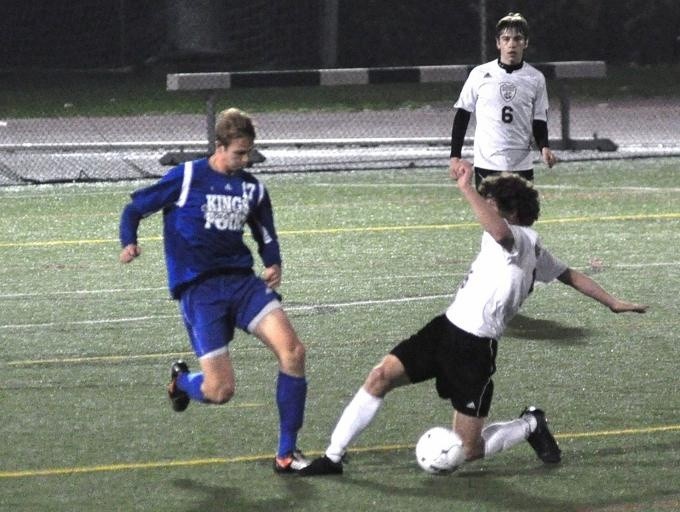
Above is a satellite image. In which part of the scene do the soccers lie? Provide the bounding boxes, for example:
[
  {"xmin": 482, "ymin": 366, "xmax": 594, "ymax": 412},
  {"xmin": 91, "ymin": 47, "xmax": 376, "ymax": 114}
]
[{"xmin": 415, "ymin": 427, "xmax": 467, "ymax": 476}]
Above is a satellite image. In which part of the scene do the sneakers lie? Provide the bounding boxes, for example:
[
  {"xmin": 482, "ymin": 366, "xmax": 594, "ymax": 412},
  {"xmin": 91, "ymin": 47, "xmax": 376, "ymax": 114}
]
[
  {"xmin": 274, "ymin": 450, "xmax": 344, "ymax": 475},
  {"xmin": 519, "ymin": 407, "xmax": 561, "ymax": 463},
  {"xmin": 168, "ymin": 361, "xmax": 189, "ymax": 412}
]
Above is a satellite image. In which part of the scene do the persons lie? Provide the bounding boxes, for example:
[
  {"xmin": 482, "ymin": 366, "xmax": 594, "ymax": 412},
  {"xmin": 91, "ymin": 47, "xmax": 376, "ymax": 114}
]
[
  {"xmin": 118, "ymin": 108, "xmax": 317, "ymax": 474},
  {"xmin": 289, "ymin": 154, "xmax": 649, "ymax": 479},
  {"xmin": 449, "ymin": 11, "xmax": 560, "ymax": 200}
]
